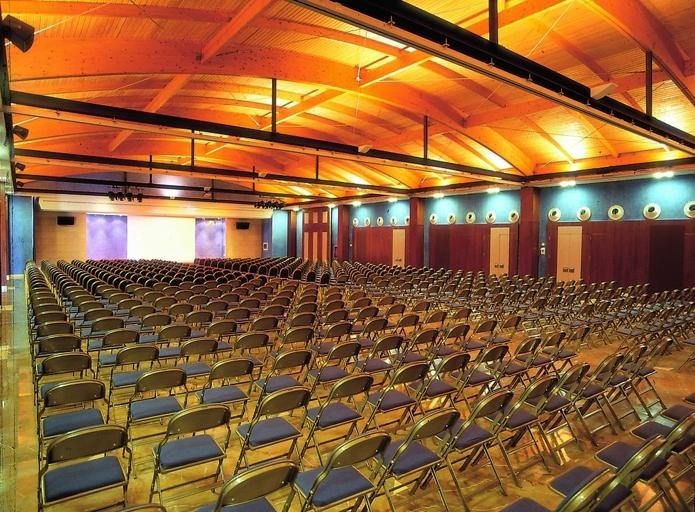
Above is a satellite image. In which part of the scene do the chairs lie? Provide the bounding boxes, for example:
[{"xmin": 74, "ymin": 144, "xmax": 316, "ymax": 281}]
[{"xmin": 25, "ymin": 256, "xmax": 695, "ymax": 512}]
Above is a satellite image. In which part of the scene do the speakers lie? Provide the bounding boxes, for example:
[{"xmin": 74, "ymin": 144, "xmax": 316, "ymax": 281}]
[
  {"xmin": 236, "ymin": 222, "xmax": 249, "ymax": 229},
  {"xmin": 57, "ymin": 216, "xmax": 74, "ymax": 225}
]
